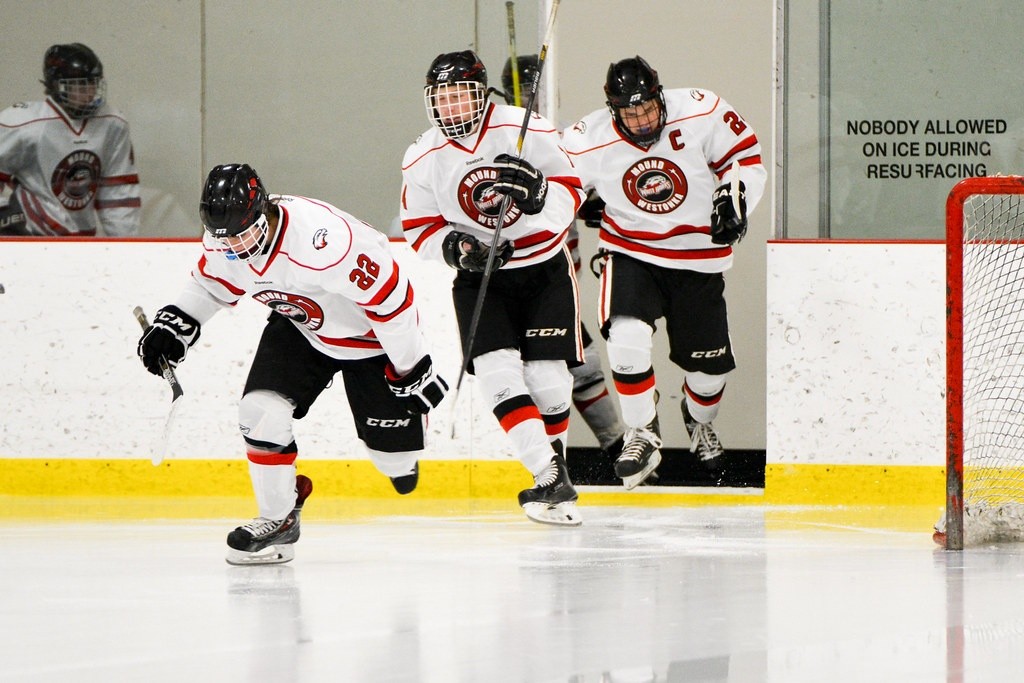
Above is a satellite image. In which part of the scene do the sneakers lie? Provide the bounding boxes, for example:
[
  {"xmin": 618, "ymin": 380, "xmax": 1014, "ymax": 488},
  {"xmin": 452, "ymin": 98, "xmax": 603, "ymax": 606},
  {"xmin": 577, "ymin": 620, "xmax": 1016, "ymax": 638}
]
[
  {"xmin": 681, "ymin": 383, "xmax": 724, "ymax": 471},
  {"xmin": 518, "ymin": 438, "xmax": 582, "ymax": 525},
  {"xmin": 225, "ymin": 474, "xmax": 313, "ymax": 564},
  {"xmin": 390, "ymin": 460, "xmax": 419, "ymax": 494},
  {"xmin": 614, "ymin": 389, "xmax": 662, "ymax": 489}
]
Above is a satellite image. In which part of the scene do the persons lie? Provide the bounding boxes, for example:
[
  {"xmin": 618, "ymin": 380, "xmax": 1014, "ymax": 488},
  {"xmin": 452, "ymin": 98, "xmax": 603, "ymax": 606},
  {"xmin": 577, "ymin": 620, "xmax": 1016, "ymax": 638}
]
[
  {"xmin": 0, "ymin": 42, "xmax": 142, "ymax": 237},
  {"xmin": 138, "ymin": 164, "xmax": 450, "ymax": 565},
  {"xmin": 501, "ymin": 54, "xmax": 539, "ymax": 111},
  {"xmin": 561, "ymin": 54, "xmax": 769, "ymax": 490},
  {"xmin": 401, "ymin": 47, "xmax": 587, "ymax": 527}
]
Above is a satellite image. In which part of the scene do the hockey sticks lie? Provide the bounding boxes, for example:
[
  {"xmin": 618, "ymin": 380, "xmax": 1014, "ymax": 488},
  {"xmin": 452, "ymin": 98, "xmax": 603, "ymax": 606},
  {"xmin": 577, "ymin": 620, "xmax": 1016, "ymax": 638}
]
[
  {"xmin": 133, "ymin": 306, "xmax": 184, "ymax": 468},
  {"xmin": 730, "ymin": 159, "xmax": 742, "ymax": 219},
  {"xmin": 445, "ymin": 0, "xmax": 560, "ymax": 440}
]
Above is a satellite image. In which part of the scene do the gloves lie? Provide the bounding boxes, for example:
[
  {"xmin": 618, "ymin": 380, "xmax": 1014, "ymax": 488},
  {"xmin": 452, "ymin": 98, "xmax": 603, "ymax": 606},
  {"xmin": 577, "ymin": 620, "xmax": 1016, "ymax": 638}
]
[
  {"xmin": 384, "ymin": 355, "xmax": 450, "ymax": 414},
  {"xmin": 137, "ymin": 304, "xmax": 201, "ymax": 377},
  {"xmin": 493, "ymin": 154, "xmax": 548, "ymax": 214},
  {"xmin": 578, "ymin": 189, "xmax": 605, "ymax": 229},
  {"xmin": 709, "ymin": 180, "xmax": 748, "ymax": 245},
  {"xmin": 442, "ymin": 230, "xmax": 514, "ymax": 272}
]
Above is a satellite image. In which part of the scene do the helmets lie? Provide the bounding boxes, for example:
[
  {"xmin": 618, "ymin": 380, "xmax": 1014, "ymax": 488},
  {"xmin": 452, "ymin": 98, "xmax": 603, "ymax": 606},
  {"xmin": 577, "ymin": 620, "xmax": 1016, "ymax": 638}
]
[
  {"xmin": 198, "ymin": 163, "xmax": 270, "ymax": 264},
  {"xmin": 42, "ymin": 43, "xmax": 107, "ymax": 118},
  {"xmin": 502, "ymin": 54, "xmax": 538, "ymax": 111},
  {"xmin": 604, "ymin": 55, "xmax": 667, "ymax": 144},
  {"xmin": 423, "ymin": 50, "xmax": 488, "ymax": 139}
]
